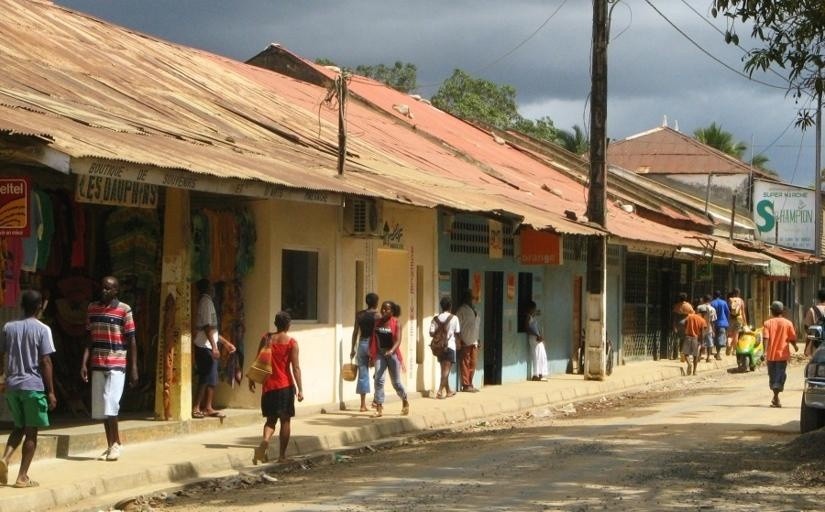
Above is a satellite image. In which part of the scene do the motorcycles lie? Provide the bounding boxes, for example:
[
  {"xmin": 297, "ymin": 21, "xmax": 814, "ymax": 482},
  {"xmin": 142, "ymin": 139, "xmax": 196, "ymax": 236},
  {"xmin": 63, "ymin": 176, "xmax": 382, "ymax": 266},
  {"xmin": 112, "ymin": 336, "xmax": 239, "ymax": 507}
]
[{"xmin": 732, "ymin": 323, "xmax": 765, "ymax": 377}]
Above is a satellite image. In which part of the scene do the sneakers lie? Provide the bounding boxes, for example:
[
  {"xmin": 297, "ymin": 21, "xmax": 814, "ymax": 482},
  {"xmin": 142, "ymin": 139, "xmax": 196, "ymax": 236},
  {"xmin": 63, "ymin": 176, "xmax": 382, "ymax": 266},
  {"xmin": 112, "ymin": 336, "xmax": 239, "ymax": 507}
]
[
  {"xmin": 97, "ymin": 442, "xmax": 121, "ymax": 461},
  {"xmin": 462, "ymin": 385, "xmax": 480, "ymax": 393}
]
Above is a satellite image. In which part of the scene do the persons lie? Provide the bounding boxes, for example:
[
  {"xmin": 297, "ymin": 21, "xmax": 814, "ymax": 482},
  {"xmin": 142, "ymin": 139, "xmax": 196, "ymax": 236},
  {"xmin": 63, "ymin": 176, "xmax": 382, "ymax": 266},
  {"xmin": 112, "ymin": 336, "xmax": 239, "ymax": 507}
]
[
  {"xmin": 670, "ymin": 283, "xmax": 825, "ymax": 407},
  {"xmin": 0, "ymin": 290, "xmax": 57, "ymax": 487},
  {"xmin": 429, "ymin": 296, "xmax": 461, "ymax": 399},
  {"xmin": 525, "ymin": 301, "xmax": 548, "ymax": 380},
  {"xmin": 349, "ymin": 292, "xmax": 381, "ymax": 412},
  {"xmin": 370, "ymin": 301, "xmax": 409, "ymax": 416},
  {"xmin": 80, "ymin": 275, "xmax": 140, "ymax": 460},
  {"xmin": 455, "ymin": 288, "xmax": 481, "ymax": 392},
  {"xmin": 192, "ymin": 279, "xmax": 237, "ymax": 417},
  {"xmin": 249, "ymin": 311, "xmax": 304, "ymax": 460}
]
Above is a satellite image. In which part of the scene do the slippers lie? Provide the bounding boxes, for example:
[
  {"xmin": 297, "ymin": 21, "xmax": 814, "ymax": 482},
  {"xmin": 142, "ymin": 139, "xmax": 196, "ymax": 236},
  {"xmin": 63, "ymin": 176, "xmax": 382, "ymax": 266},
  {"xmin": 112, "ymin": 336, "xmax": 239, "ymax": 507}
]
[
  {"xmin": 770, "ymin": 399, "xmax": 782, "ymax": 408},
  {"xmin": 436, "ymin": 390, "xmax": 456, "ymax": 399},
  {"xmin": 206, "ymin": 412, "xmax": 226, "ymax": 417},
  {"xmin": 360, "ymin": 404, "xmax": 384, "ymax": 418},
  {"xmin": 192, "ymin": 411, "xmax": 204, "ymax": 418},
  {"xmin": 401, "ymin": 401, "xmax": 410, "ymax": 416},
  {"xmin": 0, "ymin": 459, "xmax": 9, "ymax": 485},
  {"xmin": 13, "ymin": 475, "xmax": 40, "ymax": 488},
  {"xmin": 681, "ymin": 349, "xmax": 738, "ymax": 375}
]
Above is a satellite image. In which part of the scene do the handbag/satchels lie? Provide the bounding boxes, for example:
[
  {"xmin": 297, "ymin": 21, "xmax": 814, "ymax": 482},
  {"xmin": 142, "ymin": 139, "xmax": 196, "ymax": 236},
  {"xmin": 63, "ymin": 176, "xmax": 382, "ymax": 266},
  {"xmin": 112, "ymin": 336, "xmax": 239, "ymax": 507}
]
[
  {"xmin": 340, "ymin": 355, "xmax": 359, "ymax": 383},
  {"xmin": 245, "ymin": 332, "xmax": 274, "ymax": 385}
]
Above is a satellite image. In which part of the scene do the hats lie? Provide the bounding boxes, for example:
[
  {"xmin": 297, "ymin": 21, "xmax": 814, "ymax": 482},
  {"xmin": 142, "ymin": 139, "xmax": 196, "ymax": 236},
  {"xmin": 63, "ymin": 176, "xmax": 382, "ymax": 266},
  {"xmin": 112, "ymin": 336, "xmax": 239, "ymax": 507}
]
[{"xmin": 769, "ymin": 301, "xmax": 785, "ymax": 312}]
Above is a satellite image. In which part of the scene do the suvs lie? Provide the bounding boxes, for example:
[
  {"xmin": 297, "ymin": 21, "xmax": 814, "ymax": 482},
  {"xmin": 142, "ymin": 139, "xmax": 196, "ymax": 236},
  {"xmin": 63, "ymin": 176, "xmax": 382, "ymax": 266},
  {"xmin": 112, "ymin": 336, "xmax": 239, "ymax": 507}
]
[{"xmin": 797, "ymin": 324, "xmax": 825, "ymax": 437}]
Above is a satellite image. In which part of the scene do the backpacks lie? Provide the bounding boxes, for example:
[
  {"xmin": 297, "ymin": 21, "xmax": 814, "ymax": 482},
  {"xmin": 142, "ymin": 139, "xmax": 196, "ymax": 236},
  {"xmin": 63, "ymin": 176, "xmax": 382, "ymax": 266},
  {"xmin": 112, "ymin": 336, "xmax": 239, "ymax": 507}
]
[
  {"xmin": 810, "ymin": 304, "xmax": 825, "ymax": 349},
  {"xmin": 429, "ymin": 313, "xmax": 456, "ymax": 357}
]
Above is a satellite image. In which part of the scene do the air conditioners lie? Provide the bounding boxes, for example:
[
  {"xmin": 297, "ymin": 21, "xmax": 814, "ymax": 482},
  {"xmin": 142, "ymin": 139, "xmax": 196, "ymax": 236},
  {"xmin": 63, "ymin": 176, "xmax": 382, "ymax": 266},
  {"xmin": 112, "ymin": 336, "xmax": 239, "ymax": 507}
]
[{"xmin": 348, "ymin": 195, "xmax": 382, "ymax": 237}]
